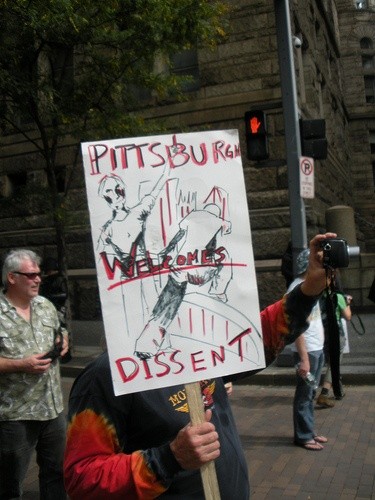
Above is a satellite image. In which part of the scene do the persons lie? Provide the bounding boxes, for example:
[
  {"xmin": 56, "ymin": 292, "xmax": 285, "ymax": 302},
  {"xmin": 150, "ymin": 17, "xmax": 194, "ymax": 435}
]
[
  {"xmin": 63, "ymin": 232, "xmax": 338, "ymax": 500},
  {"xmin": 0, "ymin": 248, "xmax": 69, "ymax": 500},
  {"xmin": 282, "ymin": 245, "xmax": 354, "ymax": 451}
]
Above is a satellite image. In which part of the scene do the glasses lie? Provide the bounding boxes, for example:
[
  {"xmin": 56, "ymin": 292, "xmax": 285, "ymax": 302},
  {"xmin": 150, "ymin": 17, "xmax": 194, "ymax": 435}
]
[{"xmin": 12, "ymin": 271, "xmax": 42, "ymax": 279}]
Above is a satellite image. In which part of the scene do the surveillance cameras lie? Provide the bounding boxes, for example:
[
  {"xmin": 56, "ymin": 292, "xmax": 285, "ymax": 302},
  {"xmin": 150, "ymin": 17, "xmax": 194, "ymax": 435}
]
[{"xmin": 294, "ymin": 38, "xmax": 301, "ymax": 48}]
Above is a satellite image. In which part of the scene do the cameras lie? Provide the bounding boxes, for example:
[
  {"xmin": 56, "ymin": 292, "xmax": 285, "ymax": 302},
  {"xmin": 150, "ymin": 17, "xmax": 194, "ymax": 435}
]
[{"xmin": 323, "ymin": 239, "xmax": 360, "ymax": 268}]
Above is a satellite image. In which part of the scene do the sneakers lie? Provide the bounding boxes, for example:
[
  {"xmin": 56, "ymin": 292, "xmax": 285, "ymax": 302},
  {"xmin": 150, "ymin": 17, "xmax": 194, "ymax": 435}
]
[{"xmin": 317, "ymin": 393, "xmax": 335, "ymax": 407}]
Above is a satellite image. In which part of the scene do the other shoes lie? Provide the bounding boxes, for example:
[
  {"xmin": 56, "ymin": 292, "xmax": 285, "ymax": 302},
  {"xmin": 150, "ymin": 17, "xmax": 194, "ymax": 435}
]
[{"xmin": 336, "ymin": 391, "xmax": 345, "ymax": 400}]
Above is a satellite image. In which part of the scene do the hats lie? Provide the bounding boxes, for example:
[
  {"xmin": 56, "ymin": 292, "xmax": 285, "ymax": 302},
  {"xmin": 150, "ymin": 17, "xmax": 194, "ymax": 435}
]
[{"xmin": 295, "ymin": 248, "xmax": 310, "ymax": 278}]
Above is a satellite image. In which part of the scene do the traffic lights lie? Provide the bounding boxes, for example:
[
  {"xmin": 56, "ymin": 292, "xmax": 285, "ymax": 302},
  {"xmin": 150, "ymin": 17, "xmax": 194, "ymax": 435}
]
[{"xmin": 243, "ymin": 108, "xmax": 272, "ymax": 161}]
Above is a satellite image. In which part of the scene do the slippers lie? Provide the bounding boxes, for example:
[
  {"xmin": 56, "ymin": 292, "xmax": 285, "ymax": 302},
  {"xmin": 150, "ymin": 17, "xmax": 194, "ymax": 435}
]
[{"xmin": 293, "ymin": 433, "xmax": 328, "ymax": 450}]
